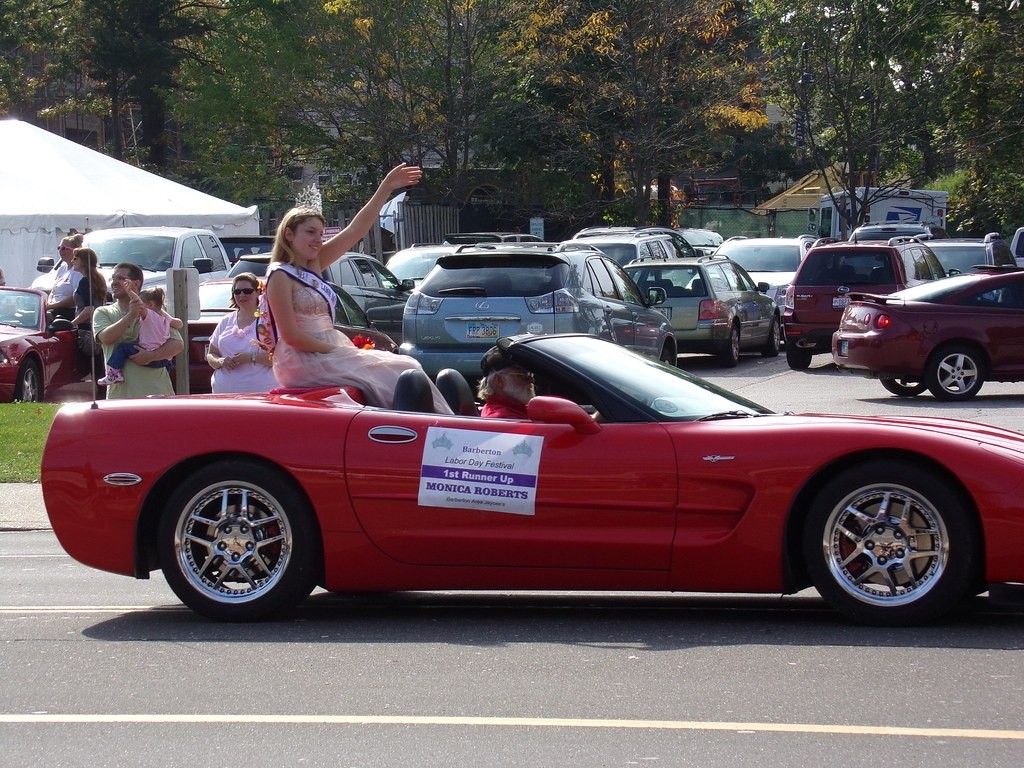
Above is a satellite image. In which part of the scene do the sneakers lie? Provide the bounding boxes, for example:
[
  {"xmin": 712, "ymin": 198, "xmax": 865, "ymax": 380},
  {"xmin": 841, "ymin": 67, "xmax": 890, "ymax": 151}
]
[{"xmin": 97, "ymin": 368, "xmax": 125, "ymax": 385}]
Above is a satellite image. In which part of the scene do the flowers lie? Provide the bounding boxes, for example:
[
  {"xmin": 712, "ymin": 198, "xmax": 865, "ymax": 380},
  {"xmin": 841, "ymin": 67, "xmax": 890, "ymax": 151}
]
[{"xmin": 354, "ymin": 334, "xmax": 376, "ymax": 350}]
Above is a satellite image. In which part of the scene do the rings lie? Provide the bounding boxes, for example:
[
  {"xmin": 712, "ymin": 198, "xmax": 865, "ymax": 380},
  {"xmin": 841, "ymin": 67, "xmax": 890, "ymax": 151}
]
[{"xmin": 238, "ymin": 362, "xmax": 240, "ymax": 365}]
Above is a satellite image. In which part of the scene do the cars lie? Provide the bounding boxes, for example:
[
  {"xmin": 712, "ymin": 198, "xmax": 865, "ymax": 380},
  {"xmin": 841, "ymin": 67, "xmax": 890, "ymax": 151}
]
[
  {"xmin": 831, "ymin": 266, "xmax": 1024, "ymax": 399},
  {"xmin": 217, "ymin": 236, "xmax": 280, "ymax": 264},
  {"xmin": 170, "ymin": 278, "xmax": 401, "ymax": 396},
  {"xmin": 229, "ymin": 251, "xmax": 414, "ymax": 348},
  {"xmin": 1010, "ymin": 227, "xmax": 1024, "ymax": 270},
  {"xmin": 677, "ymin": 229, "xmax": 723, "ymax": 253},
  {"xmin": 620, "ymin": 255, "xmax": 781, "ymax": 366},
  {"xmin": 444, "ymin": 232, "xmax": 543, "ymax": 243}
]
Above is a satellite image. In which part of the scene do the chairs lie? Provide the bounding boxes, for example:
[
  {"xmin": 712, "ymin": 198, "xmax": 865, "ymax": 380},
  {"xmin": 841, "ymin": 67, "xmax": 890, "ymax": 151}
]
[
  {"xmin": 393, "ymin": 369, "xmax": 433, "ymax": 414},
  {"xmin": 484, "ymin": 270, "xmax": 512, "ymax": 294},
  {"xmin": 659, "ymin": 279, "xmax": 676, "ymax": 298},
  {"xmin": 814, "ymin": 265, "xmax": 892, "ymax": 284},
  {"xmin": 436, "ymin": 368, "xmax": 480, "ymax": 416},
  {"xmin": 692, "ymin": 279, "xmax": 705, "ymax": 296},
  {"xmin": 24, "ymin": 311, "xmax": 54, "ymax": 326}
]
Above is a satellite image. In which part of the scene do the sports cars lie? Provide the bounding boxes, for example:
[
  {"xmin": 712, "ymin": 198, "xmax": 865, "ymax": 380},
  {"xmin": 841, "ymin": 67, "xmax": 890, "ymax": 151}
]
[
  {"xmin": 0, "ymin": 287, "xmax": 89, "ymax": 403},
  {"xmin": 41, "ymin": 332, "xmax": 1024, "ymax": 622}
]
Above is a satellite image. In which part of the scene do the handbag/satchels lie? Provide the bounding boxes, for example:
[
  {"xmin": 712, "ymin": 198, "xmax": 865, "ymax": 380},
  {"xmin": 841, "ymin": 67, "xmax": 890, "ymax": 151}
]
[{"xmin": 76, "ymin": 329, "xmax": 102, "ymax": 360}]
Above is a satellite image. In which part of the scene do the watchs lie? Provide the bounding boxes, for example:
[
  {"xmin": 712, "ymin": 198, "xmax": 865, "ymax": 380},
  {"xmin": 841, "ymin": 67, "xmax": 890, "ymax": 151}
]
[{"xmin": 251, "ymin": 352, "xmax": 256, "ymax": 366}]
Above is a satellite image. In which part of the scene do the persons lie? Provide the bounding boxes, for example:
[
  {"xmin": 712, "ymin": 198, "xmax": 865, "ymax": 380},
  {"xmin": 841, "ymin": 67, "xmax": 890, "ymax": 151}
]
[
  {"xmin": 267, "ymin": 163, "xmax": 454, "ymax": 416},
  {"xmin": 45, "ymin": 234, "xmax": 107, "ymax": 400},
  {"xmin": 93, "ymin": 262, "xmax": 184, "ymax": 400},
  {"xmin": 207, "ymin": 272, "xmax": 285, "ymax": 393},
  {"xmin": 476, "ymin": 345, "xmax": 607, "ymax": 423},
  {"xmin": 0, "ymin": 267, "xmax": 6, "ymax": 286},
  {"xmin": 98, "ymin": 278, "xmax": 183, "ymax": 385}
]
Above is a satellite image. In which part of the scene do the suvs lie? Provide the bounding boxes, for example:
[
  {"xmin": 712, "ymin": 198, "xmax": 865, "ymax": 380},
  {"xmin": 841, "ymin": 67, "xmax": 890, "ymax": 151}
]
[
  {"xmin": 909, "ymin": 232, "xmax": 1017, "ymax": 273},
  {"xmin": 401, "ymin": 240, "xmax": 678, "ymax": 389},
  {"xmin": 30, "ymin": 227, "xmax": 232, "ymax": 314},
  {"xmin": 783, "ymin": 237, "xmax": 962, "ymax": 369},
  {"xmin": 571, "ymin": 226, "xmax": 705, "ymax": 258},
  {"xmin": 686, "ymin": 236, "xmax": 825, "ymax": 315},
  {"xmin": 841, "ymin": 222, "xmax": 955, "ymax": 271}
]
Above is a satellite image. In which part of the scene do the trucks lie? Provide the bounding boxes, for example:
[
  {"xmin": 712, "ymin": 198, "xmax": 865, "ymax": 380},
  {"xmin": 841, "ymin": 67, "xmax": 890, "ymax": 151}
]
[{"xmin": 807, "ymin": 188, "xmax": 947, "ymax": 239}]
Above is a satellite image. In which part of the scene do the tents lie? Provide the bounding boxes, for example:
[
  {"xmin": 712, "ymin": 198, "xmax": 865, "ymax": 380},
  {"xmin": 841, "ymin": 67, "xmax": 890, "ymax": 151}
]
[{"xmin": 0, "ymin": 119, "xmax": 258, "ymax": 288}]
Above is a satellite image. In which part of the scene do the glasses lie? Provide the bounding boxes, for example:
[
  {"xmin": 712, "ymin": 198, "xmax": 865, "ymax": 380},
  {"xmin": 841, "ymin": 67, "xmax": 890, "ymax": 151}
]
[
  {"xmin": 233, "ymin": 288, "xmax": 256, "ymax": 296},
  {"xmin": 498, "ymin": 371, "xmax": 537, "ymax": 382},
  {"xmin": 57, "ymin": 245, "xmax": 75, "ymax": 251}
]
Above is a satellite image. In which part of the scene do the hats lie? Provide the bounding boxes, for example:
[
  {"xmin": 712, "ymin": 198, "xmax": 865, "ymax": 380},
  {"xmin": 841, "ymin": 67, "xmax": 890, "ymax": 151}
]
[{"xmin": 480, "ymin": 346, "xmax": 534, "ymax": 374}]
